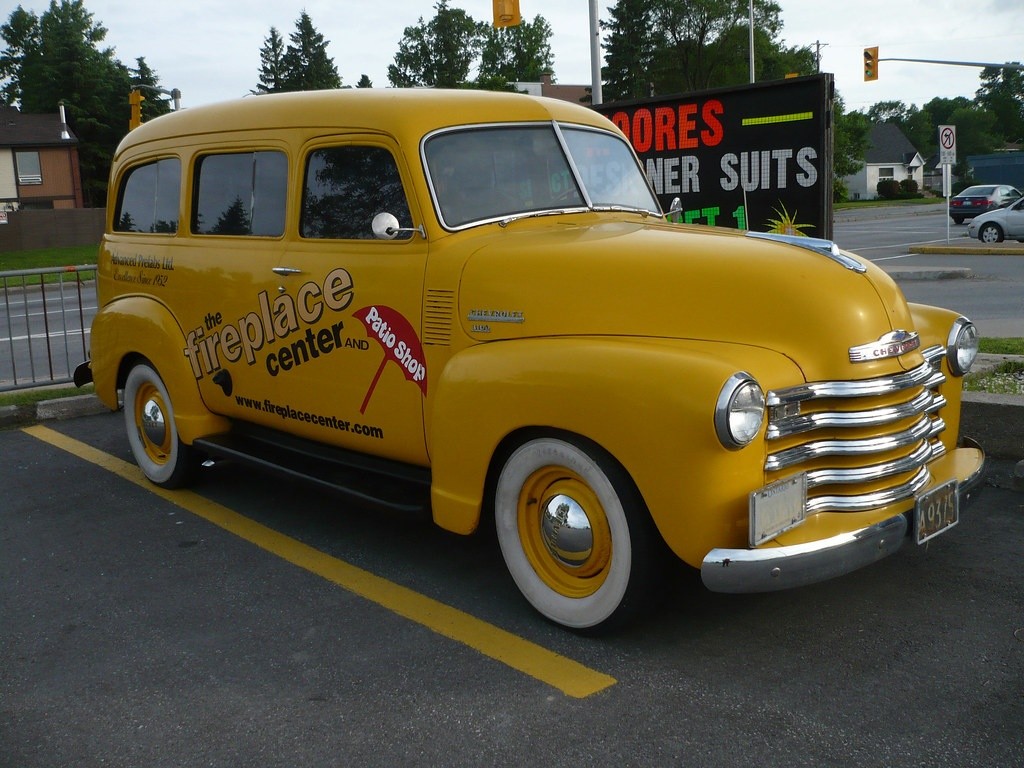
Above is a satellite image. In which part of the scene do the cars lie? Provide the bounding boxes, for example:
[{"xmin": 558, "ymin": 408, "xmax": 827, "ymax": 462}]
[
  {"xmin": 968, "ymin": 196, "xmax": 1024, "ymax": 244},
  {"xmin": 949, "ymin": 185, "xmax": 1023, "ymax": 224},
  {"xmin": 77, "ymin": 90, "xmax": 986, "ymax": 637}
]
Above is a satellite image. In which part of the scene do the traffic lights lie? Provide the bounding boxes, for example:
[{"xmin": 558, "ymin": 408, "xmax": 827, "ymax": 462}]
[
  {"xmin": 864, "ymin": 46, "xmax": 878, "ymax": 82},
  {"xmin": 129, "ymin": 89, "xmax": 146, "ymax": 131}
]
